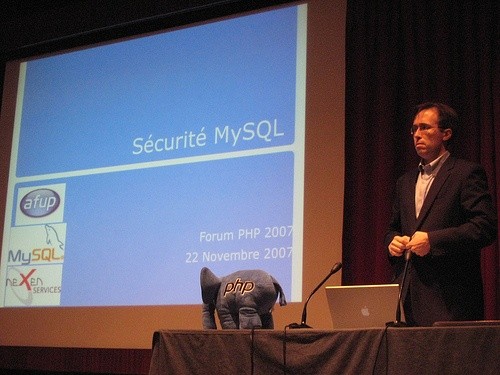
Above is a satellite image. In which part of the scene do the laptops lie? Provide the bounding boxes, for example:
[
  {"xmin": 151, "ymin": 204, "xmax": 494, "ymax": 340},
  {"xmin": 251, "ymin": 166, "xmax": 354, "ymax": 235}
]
[{"xmin": 325, "ymin": 284, "xmax": 406, "ymax": 329}]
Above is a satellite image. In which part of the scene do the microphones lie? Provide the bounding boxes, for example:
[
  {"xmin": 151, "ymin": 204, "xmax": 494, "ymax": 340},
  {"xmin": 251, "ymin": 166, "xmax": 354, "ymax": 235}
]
[
  {"xmin": 289, "ymin": 262, "xmax": 342, "ymax": 329},
  {"xmin": 386, "ymin": 250, "xmax": 412, "ymax": 328}
]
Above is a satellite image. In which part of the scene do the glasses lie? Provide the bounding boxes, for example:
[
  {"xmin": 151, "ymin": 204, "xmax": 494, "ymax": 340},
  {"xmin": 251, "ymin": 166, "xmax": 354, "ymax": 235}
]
[{"xmin": 410, "ymin": 124, "xmax": 440, "ymax": 134}]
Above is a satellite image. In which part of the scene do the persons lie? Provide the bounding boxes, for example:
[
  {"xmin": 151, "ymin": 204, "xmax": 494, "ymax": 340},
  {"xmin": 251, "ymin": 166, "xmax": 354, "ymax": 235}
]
[{"xmin": 383, "ymin": 101, "xmax": 498, "ymax": 325}]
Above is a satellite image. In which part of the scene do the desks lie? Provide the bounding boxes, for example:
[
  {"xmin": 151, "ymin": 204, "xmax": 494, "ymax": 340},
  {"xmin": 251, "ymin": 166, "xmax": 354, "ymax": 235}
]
[{"xmin": 148, "ymin": 326, "xmax": 500, "ymax": 375}]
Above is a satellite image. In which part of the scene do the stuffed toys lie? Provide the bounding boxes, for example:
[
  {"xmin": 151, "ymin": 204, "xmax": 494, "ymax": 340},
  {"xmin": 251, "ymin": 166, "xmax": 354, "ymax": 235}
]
[{"xmin": 199, "ymin": 266, "xmax": 288, "ymax": 330}]
